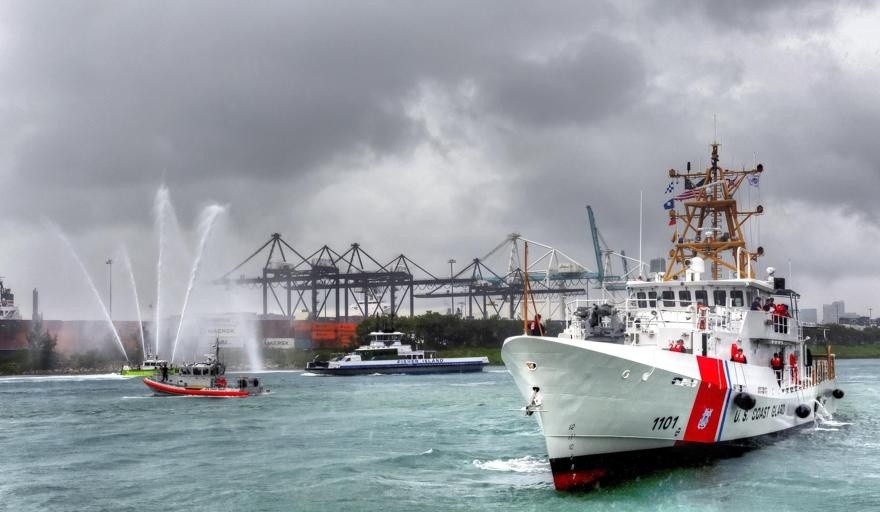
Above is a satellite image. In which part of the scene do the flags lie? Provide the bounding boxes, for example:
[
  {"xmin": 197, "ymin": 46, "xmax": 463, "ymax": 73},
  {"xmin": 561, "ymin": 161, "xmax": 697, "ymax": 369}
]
[{"xmin": 663, "ymin": 173, "xmax": 760, "ymax": 244}]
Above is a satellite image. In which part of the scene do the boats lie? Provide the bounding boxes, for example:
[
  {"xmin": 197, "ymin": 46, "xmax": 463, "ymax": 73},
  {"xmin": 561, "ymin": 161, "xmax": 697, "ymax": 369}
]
[
  {"xmin": 304, "ymin": 327, "xmax": 489, "ymax": 376},
  {"xmin": 142, "ymin": 336, "xmax": 263, "ymax": 397},
  {"xmin": 119, "ymin": 347, "xmax": 176, "ymax": 376}
]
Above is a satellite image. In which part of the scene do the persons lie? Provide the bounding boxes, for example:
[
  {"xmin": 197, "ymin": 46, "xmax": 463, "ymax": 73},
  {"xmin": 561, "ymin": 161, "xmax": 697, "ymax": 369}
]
[
  {"xmin": 158, "ymin": 364, "xmax": 170, "ymax": 384},
  {"xmin": 730, "ymin": 347, "xmax": 746, "ymax": 363},
  {"xmin": 670, "ymin": 338, "xmax": 685, "ymax": 353},
  {"xmin": 750, "ymin": 296, "xmax": 791, "ymax": 333},
  {"xmin": 771, "ymin": 352, "xmax": 783, "ymax": 388},
  {"xmin": 526, "ymin": 312, "xmax": 546, "ymax": 337}
]
